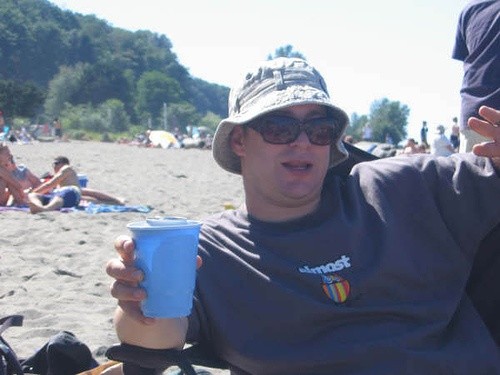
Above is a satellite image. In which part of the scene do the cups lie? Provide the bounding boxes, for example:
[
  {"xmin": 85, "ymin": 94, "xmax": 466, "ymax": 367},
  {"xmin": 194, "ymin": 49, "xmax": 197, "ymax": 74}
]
[{"xmin": 127, "ymin": 217, "xmax": 204, "ymax": 319}]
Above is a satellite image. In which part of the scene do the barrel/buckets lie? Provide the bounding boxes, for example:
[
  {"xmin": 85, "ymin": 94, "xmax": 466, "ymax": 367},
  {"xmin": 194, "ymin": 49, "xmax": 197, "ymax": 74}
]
[{"xmin": 77, "ymin": 175, "xmax": 88, "ymax": 189}]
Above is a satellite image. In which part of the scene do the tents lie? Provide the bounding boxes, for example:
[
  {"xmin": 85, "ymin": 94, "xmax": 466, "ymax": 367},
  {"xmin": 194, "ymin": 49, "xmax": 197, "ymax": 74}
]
[{"xmin": 147, "ymin": 130, "xmax": 180, "ymax": 149}]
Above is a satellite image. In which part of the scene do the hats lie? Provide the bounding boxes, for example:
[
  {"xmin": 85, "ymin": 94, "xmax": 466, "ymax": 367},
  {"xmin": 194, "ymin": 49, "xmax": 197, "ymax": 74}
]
[{"xmin": 211, "ymin": 56, "xmax": 350, "ymax": 175}]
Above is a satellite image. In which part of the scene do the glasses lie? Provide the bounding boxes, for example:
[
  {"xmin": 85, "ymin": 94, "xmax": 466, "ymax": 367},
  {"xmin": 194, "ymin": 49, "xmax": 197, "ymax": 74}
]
[
  {"xmin": 54, "ymin": 160, "xmax": 62, "ymax": 167},
  {"xmin": 239, "ymin": 114, "xmax": 345, "ymax": 146}
]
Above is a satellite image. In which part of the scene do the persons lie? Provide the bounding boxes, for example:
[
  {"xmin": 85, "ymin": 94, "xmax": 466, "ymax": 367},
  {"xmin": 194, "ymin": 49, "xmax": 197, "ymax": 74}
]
[
  {"xmin": 0, "ymin": 113, "xmax": 214, "ymax": 214},
  {"xmin": 452, "ymin": 0, "xmax": 500, "ymax": 152},
  {"xmin": 105, "ymin": 57, "xmax": 500, "ymax": 375},
  {"xmin": 342, "ymin": 117, "xmax": 460, "ymax": 158}
]
